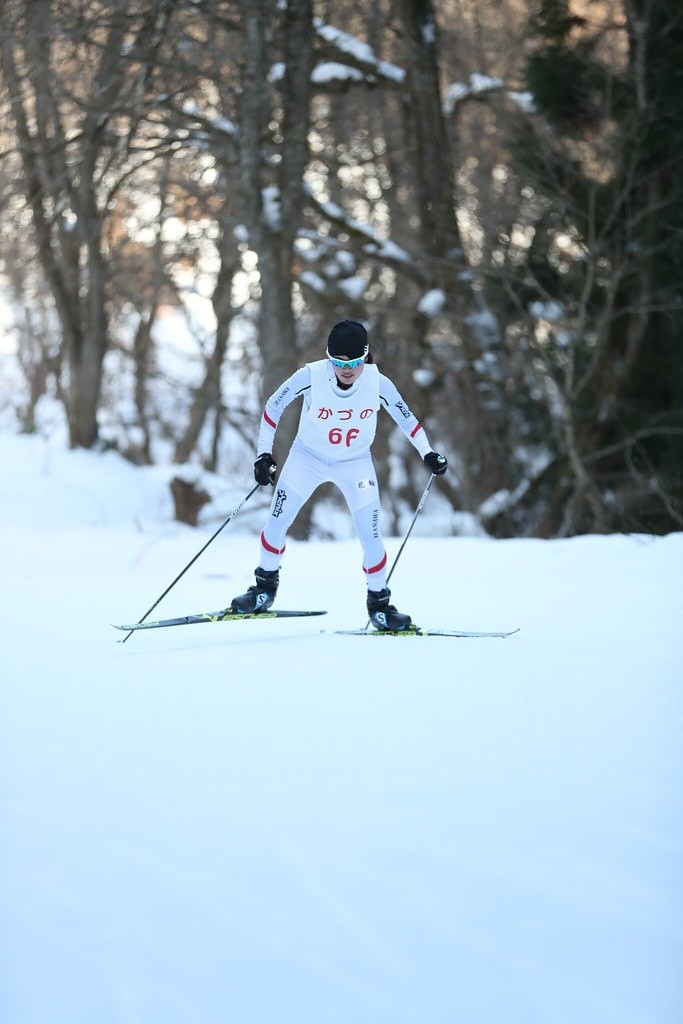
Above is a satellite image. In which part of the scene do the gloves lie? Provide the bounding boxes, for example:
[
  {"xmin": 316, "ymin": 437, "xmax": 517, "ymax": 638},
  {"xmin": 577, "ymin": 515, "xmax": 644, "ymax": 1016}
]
[
  {"xmin": 254, "ymin": 454, "xmax": 276, "ymax": 485},
  {"xmin": 425, "ymin": 452, "xmax": 448, "ymax": 475}
]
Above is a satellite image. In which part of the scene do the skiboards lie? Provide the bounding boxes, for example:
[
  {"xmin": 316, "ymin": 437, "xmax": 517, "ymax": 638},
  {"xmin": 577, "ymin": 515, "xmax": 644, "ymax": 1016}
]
[{"xmin": 111, "ymin": 608, "xmax": 524, "ymax": 639}]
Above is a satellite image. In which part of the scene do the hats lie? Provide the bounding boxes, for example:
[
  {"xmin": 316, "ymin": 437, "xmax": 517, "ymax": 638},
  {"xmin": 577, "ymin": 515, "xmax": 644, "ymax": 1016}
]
[{"xmin": 328, "ymin": 319, "xmax": 367, "ymax": 357}]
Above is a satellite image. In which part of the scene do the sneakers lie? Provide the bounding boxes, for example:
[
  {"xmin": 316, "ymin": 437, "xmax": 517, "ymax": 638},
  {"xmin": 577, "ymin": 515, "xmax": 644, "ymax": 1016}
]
[
  {"xmin": 367, "ymin": 588, "xmax": 412, "ymax": 630},
  {"xmin": 232, "ymin": 570, "xmax": 280, "ymax": 613}
]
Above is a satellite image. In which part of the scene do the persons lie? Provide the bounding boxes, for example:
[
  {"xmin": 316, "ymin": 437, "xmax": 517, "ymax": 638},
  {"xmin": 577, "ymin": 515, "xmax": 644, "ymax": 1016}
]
[{"xmin": 225, "ymin": 319, "xmax": 449, "ymax": 632}]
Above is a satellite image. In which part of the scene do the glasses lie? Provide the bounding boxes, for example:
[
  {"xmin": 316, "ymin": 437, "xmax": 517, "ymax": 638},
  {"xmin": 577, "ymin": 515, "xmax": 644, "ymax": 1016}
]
[{"xmin": 326, "ymin": 344, "xmax": 369, "ymax": 369}]
